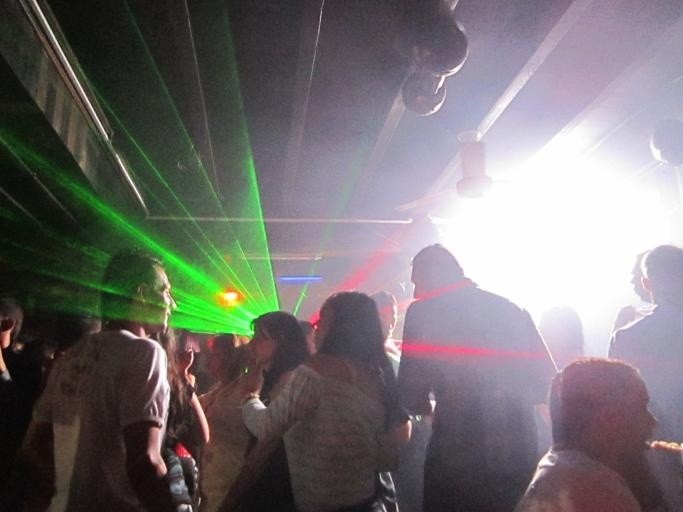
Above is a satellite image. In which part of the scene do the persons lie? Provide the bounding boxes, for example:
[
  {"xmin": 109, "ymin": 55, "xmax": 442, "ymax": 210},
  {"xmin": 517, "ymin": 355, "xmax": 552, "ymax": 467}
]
[
  {"xmin": 518, "ymin": 244, "xmax": 682, "ymax": 512},
  {"xmin": 0, "ymin": 246, "xmax": 414, "ymax": 511},
  {"xmin": 393, "ymin": 242, "xmax": 558, "ymax": 511}
]
